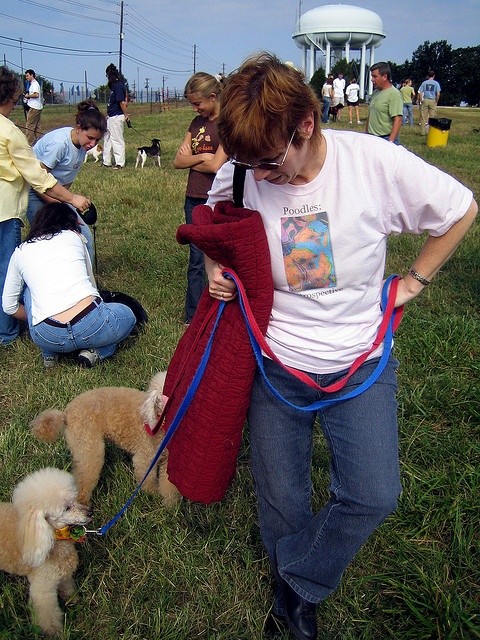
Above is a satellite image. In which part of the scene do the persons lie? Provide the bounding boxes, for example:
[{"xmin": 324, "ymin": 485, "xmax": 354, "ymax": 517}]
[
  {"xmin": 27, "ymin": 100, "xmax": 107, "ymax": 263},
  {"xmin": 333, "ymin": 73, "xmax": 345, "ymax": 122},
  {"xmin": 400, "ymin": 79, "xmax": 415, "ymax": 127},
  {"xmin": 320, "ymin": 77, "xmax": 335, "ymax": 123},
  {"xmin": 345, "ymin": 78, "xmax": 364, "ymax": 125},
  {"xmin": 0, "ymin": 66, "xmax": 92, "ymax": 343},
  {"xmin": 2, "ymin": 201, "xmax": 136, "ymax": 368},
  {"xmin": 366, "ymin": 62, "xmax": 403, "ymax": 145},
  {"xmin": 21, "ymin": 69, "xmax": 43, "ymax": 145},
  {"xmin": 204, "ymin": 49, "xmax": 478, "ymax": 639},
  {"xmin": 173, "ymin": 72, "xmax": 230, "ymax": 323},
  {"xmin": 419, "ymin": 71, "xmax": 441, "ymax": 136},
  {"xmin": 99, "ymin": 63, "xmax": 130, "ymax": 170}
]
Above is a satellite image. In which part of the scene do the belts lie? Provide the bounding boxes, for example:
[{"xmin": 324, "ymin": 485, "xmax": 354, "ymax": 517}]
[{"xmin": 43, "ymin": 297, "xmax": 102, "ymax": 328}]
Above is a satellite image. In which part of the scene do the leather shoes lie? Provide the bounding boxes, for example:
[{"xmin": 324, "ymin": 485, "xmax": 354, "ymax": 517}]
[
  {"xmin": 260, "ymin": 614, "xmax": 286, "ymax": 640},
  {"xmin": 273, "ymin": 550, "xmax": 318, "ymax": 640}
]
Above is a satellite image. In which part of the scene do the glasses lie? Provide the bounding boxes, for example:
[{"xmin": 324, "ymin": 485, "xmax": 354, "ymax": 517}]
[{"xmin": 230, "ymin": 126, "xmax": 299, "ymax": 170}]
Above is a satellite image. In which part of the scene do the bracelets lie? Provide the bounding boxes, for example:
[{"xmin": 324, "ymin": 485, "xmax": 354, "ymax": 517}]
[{"xmin": 407, "ymin": 267, "xmax": 430, "ymax": 287}]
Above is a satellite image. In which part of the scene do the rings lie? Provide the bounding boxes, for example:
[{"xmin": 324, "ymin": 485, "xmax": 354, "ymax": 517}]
[{"xmin": 222, "ymin": 292, "xmax": 224, "ymax": 296}]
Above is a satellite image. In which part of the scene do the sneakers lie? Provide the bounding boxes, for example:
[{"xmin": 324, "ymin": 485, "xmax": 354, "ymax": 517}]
[
  {"xmin": 77, "ymin": 348, "xmax": 99, "ymax": 367},
  {"xmin": 349, "ymin": 121, "xmax": 352, "ymax": 124},
  {"xmin": 44, "ymin": 356, "xmax": 56, "ymax": 368},
  {"xmin": 356, "ymin": 122, "xmax": 363, "ymax": 125}
]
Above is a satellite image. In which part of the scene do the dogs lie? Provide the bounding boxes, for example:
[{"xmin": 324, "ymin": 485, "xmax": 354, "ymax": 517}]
[
  {"xmin": 321, "ymin": 103, "xmax": 345, "ymax": 122},
  {"xmin": 82, "ymin": 143, "xmax": 103, "ymax": 163},
  {"xmin": 135, "ymin": 138, "xmax": 162, "ymax": 169},
  {"xmin": 29, "ymin": 370, "xmax": 182, "ymax": 514},
  {"xmin": 1, "ymin": 467, "xmax": 90, "ymax": 640}
]
